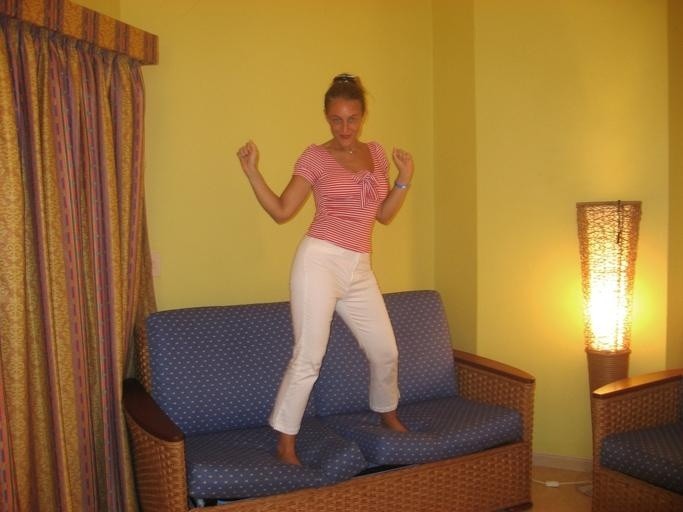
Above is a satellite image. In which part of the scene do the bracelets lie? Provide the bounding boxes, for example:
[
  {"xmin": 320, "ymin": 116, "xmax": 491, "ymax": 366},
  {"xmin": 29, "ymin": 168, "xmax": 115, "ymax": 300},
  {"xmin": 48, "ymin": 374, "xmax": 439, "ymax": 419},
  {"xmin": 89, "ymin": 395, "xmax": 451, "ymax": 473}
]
[{"xmin": 393, "ymin": 178, "xmax": 411, "ymax": 193}]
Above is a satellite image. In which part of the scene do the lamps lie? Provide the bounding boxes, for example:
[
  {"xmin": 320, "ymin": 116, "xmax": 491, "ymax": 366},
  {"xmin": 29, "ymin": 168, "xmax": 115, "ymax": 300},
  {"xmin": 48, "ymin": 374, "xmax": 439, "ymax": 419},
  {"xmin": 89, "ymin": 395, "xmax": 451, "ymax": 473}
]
[{"xmin": 573, "ymin": 200, "xmax": 640, "ymax": 391}]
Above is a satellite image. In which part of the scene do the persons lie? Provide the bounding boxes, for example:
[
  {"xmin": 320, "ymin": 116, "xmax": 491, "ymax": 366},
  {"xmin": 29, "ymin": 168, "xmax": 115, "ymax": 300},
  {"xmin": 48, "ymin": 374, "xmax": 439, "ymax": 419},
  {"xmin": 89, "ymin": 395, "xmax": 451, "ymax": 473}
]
[{"xmin": 235, "ymin": 71, "xmax": 415, "ymax": 469}]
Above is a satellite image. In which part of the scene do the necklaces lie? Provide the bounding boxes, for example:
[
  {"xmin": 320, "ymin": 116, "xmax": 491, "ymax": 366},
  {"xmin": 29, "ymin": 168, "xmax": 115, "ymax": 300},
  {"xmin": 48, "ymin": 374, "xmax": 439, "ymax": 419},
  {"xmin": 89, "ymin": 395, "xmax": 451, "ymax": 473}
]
[{"xmin": 332, "ymin": 137, "xmax": 359, "ymax": 157}]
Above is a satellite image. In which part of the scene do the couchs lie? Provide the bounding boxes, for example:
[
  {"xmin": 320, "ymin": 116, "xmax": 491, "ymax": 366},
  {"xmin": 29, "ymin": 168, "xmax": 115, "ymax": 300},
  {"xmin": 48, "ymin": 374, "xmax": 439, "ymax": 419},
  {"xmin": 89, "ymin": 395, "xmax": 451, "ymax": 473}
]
[
  {"xmin": 111, "ymin": 292, "xmax": 536, "ymax": 512},
  {"xmin": 589, "ymin": 366, "xmax": 683, "ymax": 511}
]
